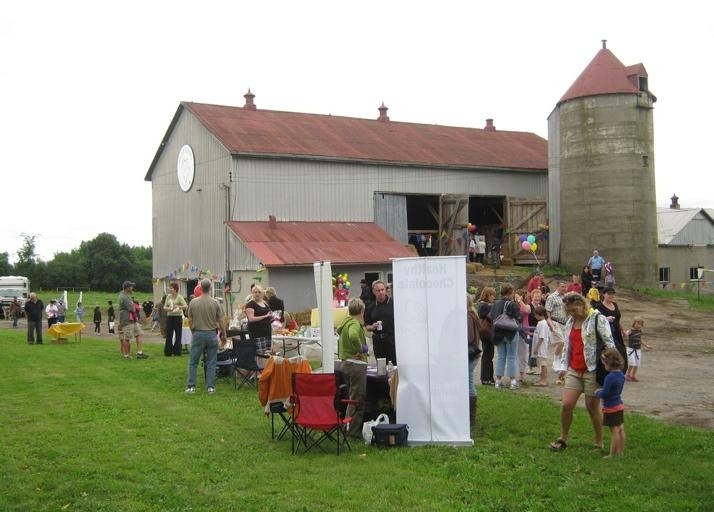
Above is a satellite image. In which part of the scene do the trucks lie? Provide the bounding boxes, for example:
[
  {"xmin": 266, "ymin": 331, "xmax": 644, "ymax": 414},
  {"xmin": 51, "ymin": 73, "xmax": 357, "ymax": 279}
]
[{"xmin": 0, "ymin": 275, "xmax": 29, "ymax": 317}]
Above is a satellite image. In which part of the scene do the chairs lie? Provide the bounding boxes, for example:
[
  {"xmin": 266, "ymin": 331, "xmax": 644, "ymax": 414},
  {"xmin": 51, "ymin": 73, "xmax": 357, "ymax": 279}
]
[{"xmin": 203, "ymin": 339, "xmax": 359, "ymax": 454}]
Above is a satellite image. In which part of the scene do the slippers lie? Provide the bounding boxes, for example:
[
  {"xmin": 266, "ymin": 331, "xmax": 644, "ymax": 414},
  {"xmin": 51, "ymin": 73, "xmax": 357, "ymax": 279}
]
[{"xmin": 548, "ymin": 439, "xmax": 567, "ymax": 450}]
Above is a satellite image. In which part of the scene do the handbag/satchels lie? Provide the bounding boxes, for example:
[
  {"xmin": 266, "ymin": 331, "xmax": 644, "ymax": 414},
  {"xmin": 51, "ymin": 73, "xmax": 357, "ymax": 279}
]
[
  {"xmin": 469, "ymin": 342, "xmax": 482, "ymax": 360},
  {"xmin": 596, "ymin": 337, "xmax": 608, "ymax": 386},
  {"xmin": 479, "ymin": 319, "xmax": 493, "ymax": 335},
  {"xmin": 493, "ymin": 314, "xmax": 518, "ymax": 332},
  {"xmin": 362, "ymin": 414, "xmax": 389, "ymax": 445}
]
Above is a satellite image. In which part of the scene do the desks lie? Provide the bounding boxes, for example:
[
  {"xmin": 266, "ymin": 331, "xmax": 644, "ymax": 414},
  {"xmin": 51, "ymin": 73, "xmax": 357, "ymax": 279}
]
[
  {"xmin": 172, "ymin": 328, "xmax": 192, "ymax": 354},
  {"xmin": 48, "ymin": 323, "xmax": 85, "ymax": 344},
  {"xmin": 334, "ymin": 359, "xmax": 397, "ymax": 436}
]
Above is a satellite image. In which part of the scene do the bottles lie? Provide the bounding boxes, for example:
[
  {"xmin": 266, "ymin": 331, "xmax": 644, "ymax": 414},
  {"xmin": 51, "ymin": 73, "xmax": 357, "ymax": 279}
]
[{"xmin": 387, "ymin": 360, "xmax": 393, "ymax": 376}]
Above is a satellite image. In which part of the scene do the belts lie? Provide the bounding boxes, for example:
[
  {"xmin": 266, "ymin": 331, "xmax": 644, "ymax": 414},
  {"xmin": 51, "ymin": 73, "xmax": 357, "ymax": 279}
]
[{"xmin": 552, "ymin": 318, "xmax": 565, "ymax": 325}]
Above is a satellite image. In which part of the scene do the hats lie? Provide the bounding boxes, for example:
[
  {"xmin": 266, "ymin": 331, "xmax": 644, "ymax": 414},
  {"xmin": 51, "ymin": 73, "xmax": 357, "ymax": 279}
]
[
  {"xmin": 604, "ymin": 262, "xmax": 612, "ymax": 272},
  {"xmin": 603, "ymin": 287, "xmax": 616, "ymax": 293},
  {"xmin": 122, "ymin": 281, "xmax": 136, "ymax": 288}
]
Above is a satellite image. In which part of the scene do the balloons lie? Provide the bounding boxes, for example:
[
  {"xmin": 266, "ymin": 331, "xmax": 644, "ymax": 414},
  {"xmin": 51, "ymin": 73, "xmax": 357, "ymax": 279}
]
[
  {"xmin": 519, "ymin": 234, "xmax": 537, "ymax": 252},
  {"xmin": 468, "ymin": 224, "xmax": 476, "ymax": 231}
]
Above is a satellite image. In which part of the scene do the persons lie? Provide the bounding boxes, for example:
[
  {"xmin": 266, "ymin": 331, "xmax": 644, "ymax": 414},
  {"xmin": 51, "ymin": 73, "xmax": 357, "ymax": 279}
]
[
  {"xmin": 588, "ymin": 249, "xmax": 605, "ymax": 282},
  {"xmin": 548, "ymin": 291, "xmax": 616, "ymax": 450},
  {"xmin": 469, "ymin": 234, "xmax": 486, "ymax": 265},
  {"xmin": 477, "ymin": 275, "xmax": 601, "ymax": 390},
  {"xmin": 57, "ymin": 300, "xmax": 66, "ymax": 323},
  {"xmin": 594, "ymin": 348, "xmax": 626, "ymax": 457},
  {"xmin": 24, "ymin": 292, "xmax": 45, "ymax": 345},
  {"xmin": 333, "ymin": 271, "xmax": 397, "ymax": 439},
  {"xmin": 107, "ymin": 300, "xmax": 116, "ymax": 335},
  {"xmin": 581, "ymin": 265, "xmax": 595, "ymax": 297},
  {"xmin": 409, "ymin": 232, "xmax": 438, "ymax": 256},
  {"xmin": 46, "ymin": 300, "xmax": 58, "ymax": 328},
  {"xmin": 93, "ymin": 306, "xmax": 102, "ymax": 336},
  {"xmin": 595, "ymin": 288, "xmax": 627, "ymax": 375},
  {"xmin": 118, "ymin": 277, "xmax": 285, "ymax": 395},
  {"xmin": 623, "ymin": 318, "xmax": 651, "ymax": 382},
  {"xmin": 9, "ymin": 296, "xmax": 23, "ymax": 329},
  {"xmin": 468, "ymin": 293, "xmax": 479, "ymax": 437},
  {"xmin": 74, "ymin": 302, "xmax": 86, "ymax": 332}
]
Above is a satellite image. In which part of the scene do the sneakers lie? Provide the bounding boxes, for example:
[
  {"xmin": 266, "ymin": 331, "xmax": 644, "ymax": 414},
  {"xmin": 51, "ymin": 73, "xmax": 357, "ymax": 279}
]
[
  {"xmin": 137, "ymin": 353, "xmax": 148, "ymax": 359},
  {"xmin": 208, "ymin": 387, "xmax": 215, "ymax": 394},
  {"xmin": 482, "ymin": 379, "xmax": 521, "ymax": 390},
  {"xmin": 186, "ymin": 387, "xmax": 195, "ymax": 393}
]
